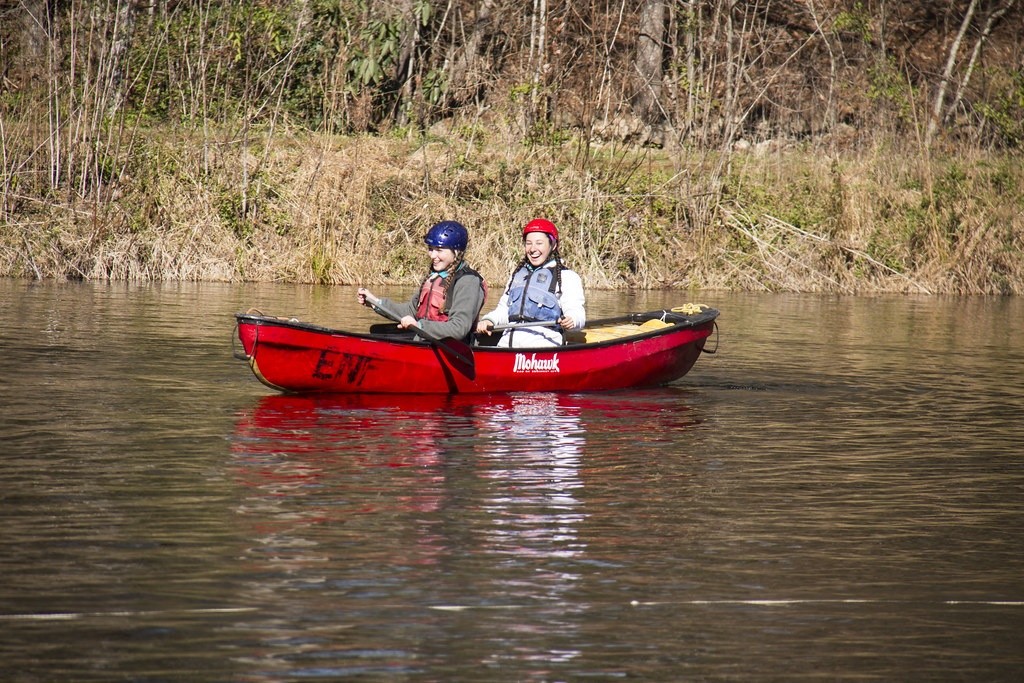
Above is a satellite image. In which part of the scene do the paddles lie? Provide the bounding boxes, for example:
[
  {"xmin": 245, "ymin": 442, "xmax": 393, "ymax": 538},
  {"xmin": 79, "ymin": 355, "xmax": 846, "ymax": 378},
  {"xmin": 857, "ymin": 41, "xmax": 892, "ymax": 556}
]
[
  {"xmin": 370, "ymin": 315, "xmax": 565, "ymax": 337},
  {"xmin": 359, "ymin": 286, "xmax": 475, "ymax": 381}
]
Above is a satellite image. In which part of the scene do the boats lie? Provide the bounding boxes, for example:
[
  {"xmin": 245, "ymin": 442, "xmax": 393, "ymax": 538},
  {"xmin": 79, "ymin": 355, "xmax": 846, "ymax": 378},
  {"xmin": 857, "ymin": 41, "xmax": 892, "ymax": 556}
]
[{"xmin": 230, "ymin": 304, "xmax": 722, "ymax": 398}]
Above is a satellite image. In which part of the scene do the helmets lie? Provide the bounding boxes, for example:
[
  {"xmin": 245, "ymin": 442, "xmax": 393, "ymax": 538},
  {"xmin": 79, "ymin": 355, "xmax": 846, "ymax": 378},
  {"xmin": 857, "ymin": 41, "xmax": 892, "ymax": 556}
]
[
  {"xmin": 425, "ymin": 221, "xmax": 468, "ymax": 251},
  {"xmin": 523, "ymin": 218, "xmax": 558, "ymax": 246}
]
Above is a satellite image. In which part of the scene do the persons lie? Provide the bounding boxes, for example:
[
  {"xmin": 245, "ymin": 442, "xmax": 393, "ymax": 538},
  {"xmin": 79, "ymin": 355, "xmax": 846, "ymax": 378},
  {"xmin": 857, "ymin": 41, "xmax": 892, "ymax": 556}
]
[
  {"xmin": 475, "ymin": 218, "xmax": 586, "ymax": 348},
  {"xmin": 356, "ymin": 220, "xmax": 488, "ymax": 344}
]
